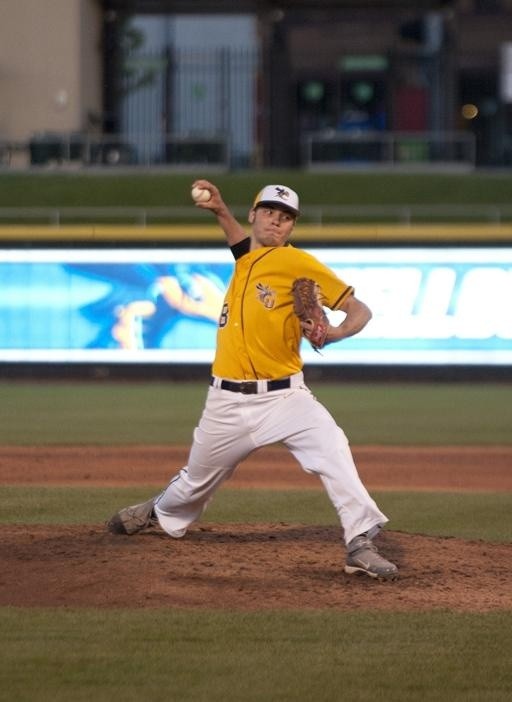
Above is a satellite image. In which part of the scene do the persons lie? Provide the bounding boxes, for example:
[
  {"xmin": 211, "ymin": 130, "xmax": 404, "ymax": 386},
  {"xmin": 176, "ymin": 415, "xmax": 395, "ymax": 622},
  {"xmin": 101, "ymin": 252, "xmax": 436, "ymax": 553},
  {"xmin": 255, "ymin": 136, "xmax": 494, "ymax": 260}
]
[{"xmin": 104, "ymin": 178, "xmax": 402, "ymax": 585}]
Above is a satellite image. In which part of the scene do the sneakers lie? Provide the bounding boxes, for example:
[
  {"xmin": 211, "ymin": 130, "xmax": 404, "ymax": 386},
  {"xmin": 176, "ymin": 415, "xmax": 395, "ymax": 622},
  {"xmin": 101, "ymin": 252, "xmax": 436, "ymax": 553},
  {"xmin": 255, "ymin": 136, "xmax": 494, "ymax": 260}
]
[
  {"xmin": 108, "ymin": 498, "xmax": 158, "ymax": 537},
  {"xmin": 345, "ymin": 534, "xmax": 399, "ymax": 578}
]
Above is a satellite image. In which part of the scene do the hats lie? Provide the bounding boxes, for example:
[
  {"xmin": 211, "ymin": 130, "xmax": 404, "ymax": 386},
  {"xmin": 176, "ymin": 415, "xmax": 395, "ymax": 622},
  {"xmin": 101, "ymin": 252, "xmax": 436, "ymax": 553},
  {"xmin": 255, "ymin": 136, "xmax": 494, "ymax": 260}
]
[{"xmin": 254, "ymin": 184, "xmax": 301, "ymax": 216}]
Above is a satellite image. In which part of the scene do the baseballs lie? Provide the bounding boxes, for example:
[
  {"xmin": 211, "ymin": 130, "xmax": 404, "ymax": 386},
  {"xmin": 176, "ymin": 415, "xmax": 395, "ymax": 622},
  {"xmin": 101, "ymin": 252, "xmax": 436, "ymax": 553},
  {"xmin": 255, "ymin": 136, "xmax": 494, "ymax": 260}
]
[
  {"xmin": 191, "ymin": 186, "xmax": 211, "ymax": 202},
  {"xmin": 463, "ymin": 104, "xmax": 476, "ymax": 118}
]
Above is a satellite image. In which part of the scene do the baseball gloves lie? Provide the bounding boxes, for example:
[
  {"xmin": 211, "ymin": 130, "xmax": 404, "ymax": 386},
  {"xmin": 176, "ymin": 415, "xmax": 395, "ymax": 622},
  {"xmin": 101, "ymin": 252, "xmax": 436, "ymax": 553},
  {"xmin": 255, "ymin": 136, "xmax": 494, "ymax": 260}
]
[{"xmin": 294, "ymin": 278, "xmax": 328, "ymax": 349}]
